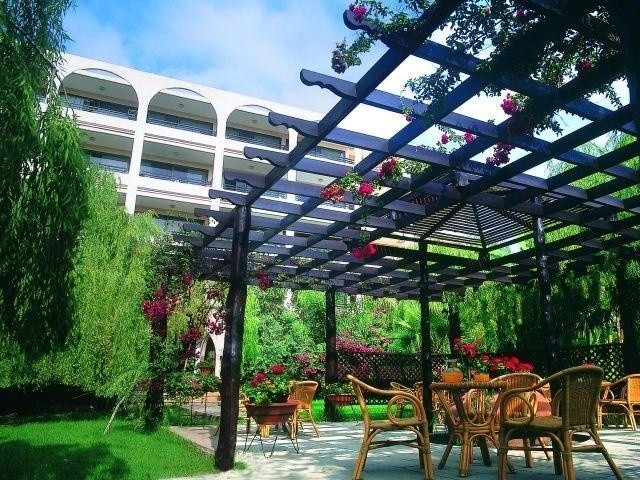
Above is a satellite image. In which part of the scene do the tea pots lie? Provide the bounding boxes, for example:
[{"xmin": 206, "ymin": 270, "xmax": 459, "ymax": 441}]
[{"xmin": 441, "ymin": 358, "xmax": 463, "ymax": 383}]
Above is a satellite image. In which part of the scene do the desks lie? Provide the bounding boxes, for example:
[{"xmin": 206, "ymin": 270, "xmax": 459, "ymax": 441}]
[{"xmin": 429, "ymin": 382, "xmax": 517, "ymax": 475}]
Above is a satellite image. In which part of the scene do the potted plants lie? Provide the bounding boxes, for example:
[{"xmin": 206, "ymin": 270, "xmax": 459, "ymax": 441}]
[
  {"xmin": 322, "ymin": 384, "xmax": 361, "ymax": 424},
  {"xmin": 245, "ymin": 402, "xmax": 301, "ymax": 458}
]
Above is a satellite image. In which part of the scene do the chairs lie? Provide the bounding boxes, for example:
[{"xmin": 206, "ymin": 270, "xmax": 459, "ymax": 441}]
[
  {"xmin": 497, "ymin": 366, "xmax": 621, "ymax": 479},
  {"xmin": 459, "ymin": 373, "xmax": 551, "ymax": 478},
  {"xmin": 390, "ymin": 381, "xmax": 415, "ymax": 422},
  {"xmin": 412, "ymin": 380, "xmax": 436, "ymax": 412},
  {"xmin": 346, "ymin": 374, "xmax": 434, "ymax": 480},
  {"xmin": 597, "ymin": 374, "xmax": 640, "ymax": 431},
  {"xmin": 249, "ymin": 382, "xmax": 321, "ymax": 436}
]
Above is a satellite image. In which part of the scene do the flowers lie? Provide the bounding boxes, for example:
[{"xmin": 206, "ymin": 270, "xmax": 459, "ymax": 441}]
[
  {"xmin": 452, "ymin": 336, "xmax": 519, "ymax": 364},
  {"xmin": 298, "ymin": 351, "xmax": 326, "ymax": 381},
  {"xmin": 252, "ymin": 366, "xmax": 288, "ymax": 387}
]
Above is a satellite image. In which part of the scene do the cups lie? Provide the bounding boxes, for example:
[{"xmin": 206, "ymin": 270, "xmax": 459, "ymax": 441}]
[{"xmin": 474, "ymin": 372, "xmax": 490, "ymax": 382}]
